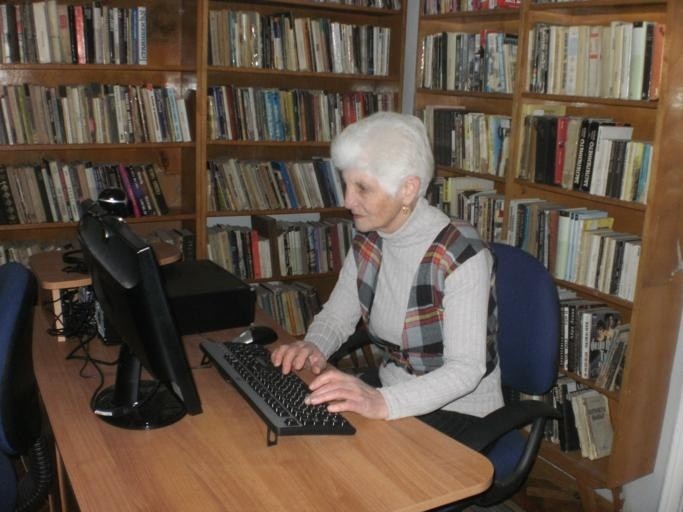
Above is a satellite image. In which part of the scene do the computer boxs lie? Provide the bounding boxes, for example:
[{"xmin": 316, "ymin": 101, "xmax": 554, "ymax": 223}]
[{"xmin": 78, "ymin": 260, "xmax": 254, "ymax": 345}]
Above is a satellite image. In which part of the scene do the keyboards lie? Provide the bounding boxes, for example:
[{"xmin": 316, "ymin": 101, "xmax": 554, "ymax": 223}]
[{"xmin": 199, "ymin": 340, "xmax": 356, "ymax": 446}]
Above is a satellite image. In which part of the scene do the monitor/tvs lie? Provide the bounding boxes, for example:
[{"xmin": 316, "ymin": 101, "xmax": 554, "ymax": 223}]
[{"xmin": 78, "ymin": 200, "xmax": 203, "ymax": 430}]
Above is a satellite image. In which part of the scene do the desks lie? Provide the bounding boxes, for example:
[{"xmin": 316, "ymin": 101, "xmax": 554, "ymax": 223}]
[{"xmin": 26, "ymin": 241, "xmax": 497, "ymax": 512}]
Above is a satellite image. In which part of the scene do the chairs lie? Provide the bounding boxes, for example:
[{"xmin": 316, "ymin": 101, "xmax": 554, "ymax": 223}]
[
  {"xmin": 0, "ymin": 259, "xmax": 50, "ymax": 512},
  {"xmin": 325, "ymin": 241, "xmax": 564, "ymax": 511}
]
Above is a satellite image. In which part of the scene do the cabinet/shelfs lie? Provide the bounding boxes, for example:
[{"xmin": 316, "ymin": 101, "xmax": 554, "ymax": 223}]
[
  {"xmin": 509, "ymin": 1, "xmax": 683, "ymax": 511},
  {"xmin": 202, "ymin": 0, "xmax": 410, "ymax": 343},
  {"xmin": 0, "ymin": 1, "xmax": 202, "ymax": 272},
  {"xmin": 411, "ymin": 1, "xmax": 523, "ymax": 243}
]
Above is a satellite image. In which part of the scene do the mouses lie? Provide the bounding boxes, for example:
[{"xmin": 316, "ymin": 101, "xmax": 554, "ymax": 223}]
[{"xmin": 234, "ymin": 324, "xmax": 277, "ymax": 346}]
